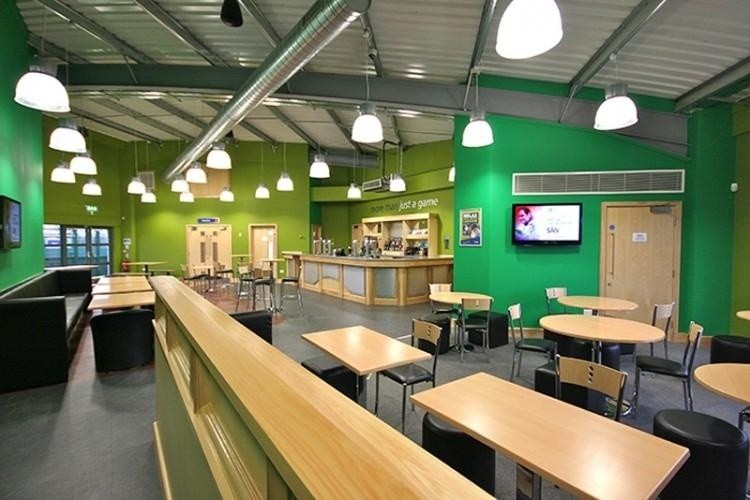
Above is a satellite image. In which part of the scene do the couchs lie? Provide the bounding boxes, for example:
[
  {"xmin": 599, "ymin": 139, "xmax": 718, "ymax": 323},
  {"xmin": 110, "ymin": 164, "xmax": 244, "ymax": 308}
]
[
  {"xmin": 2, "ymin": 263, "xmax": 94, "ymax": 394},
  {"xmin": 229, "ymin": 309, "xmax": 364, "ymax": 402}
]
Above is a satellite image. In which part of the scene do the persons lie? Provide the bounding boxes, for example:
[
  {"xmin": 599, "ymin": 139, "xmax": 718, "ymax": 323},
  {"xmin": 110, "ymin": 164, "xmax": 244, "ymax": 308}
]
[
  {"xmin": 517, "ymin": 208, "xmax": 538, "ymax": 240},
  {"xmin": 471, "ymin": 223, "xmax": 480, "ymax": 237}
]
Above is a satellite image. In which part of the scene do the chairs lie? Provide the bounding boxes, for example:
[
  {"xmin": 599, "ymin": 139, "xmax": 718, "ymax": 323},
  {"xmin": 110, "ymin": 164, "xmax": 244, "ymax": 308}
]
[
  {"xmin": 554, "ymin": 355, "xmax": 627, "ymax": 422},
  {"xmin": 417, "ymin": 284, "xmax": 510, "ymax": 363},
  {"xmin": 375, "ymin": 317, "xmax": 443, "ymax": 434},
  {"xmin": 633, "ymin": 320, "xmax": 704, "ymax": 407},
  {"xmin": 184, "ymin": 258, "xmax": 304, "ymax": 313},
  {"xmin": 539, "ymin": 286, "xmax": 675, "ymax": 361},
  {"xmin": 509, "ymin": 304, "xmax": 558, "ymax": 380}
]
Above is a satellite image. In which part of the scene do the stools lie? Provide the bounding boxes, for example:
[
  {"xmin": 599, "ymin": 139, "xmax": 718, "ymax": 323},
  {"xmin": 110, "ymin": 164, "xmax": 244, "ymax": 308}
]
[
  {"xmin": 654, "ymin": 408, "xmax": 749, "ymax": 499},
  {"xmin": 421, "ymin": 411, "xmax": 496, "ymax": 496},
  {"xmin": 709, "ymin": 336, "xmax": 750, "ymax": 366}
]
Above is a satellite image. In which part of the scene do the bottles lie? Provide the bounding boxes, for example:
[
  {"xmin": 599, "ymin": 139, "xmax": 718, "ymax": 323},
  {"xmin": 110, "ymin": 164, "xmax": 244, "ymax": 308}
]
[{"xmin": 333, "ymin": 249, "xmax": 336, "ymax": 256}]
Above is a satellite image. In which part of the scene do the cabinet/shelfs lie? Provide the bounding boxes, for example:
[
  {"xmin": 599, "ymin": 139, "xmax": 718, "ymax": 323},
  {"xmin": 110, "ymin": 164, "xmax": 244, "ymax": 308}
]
[{"xmin": 360, "ymin": 212, "xmax": 440, "ymax": 255}]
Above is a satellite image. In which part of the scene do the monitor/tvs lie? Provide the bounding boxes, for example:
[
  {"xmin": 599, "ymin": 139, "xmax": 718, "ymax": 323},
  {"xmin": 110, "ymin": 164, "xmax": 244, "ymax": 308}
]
[
  {"xmin": 0, "ymin": 194, "xmax": 21, "ymax": 250},
  {"xmin": 336, "ymin": 252, "xmax": 346, "ymax": 256},
  {"xmin": 512, "ymin": 203, "xmax": 582, "ymax": 246}
]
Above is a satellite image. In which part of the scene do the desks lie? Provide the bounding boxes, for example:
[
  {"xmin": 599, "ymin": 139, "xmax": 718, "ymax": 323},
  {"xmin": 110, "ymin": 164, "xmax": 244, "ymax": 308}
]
[
  {"xmin": 407, "ymin": 372, "xmax": 691, "ymax": 500},
  {"xmin": 738, "ymin": 309, "xmax": 748, "ymax": 319},
  {"xmin": 301, "ymin": 318, "xmax": 434, "ymax": 403},
  {"xmin": 87, "ymin": 261, "xmax": 177, "ymax": 375},
  {"xmin": 694, "ymin": 362, "xmax": 750, "ymax": 432}
]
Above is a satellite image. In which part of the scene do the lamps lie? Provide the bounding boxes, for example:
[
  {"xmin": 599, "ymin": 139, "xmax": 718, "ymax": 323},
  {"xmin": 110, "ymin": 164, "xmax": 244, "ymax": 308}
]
[
  {"xmin": 591, "ymin": 49, "xmax": 641, "ymax": 135},
  {"xmin": 459, "ymin": 64, "xmax": 500, "ymax": 151},
  {"xmin": 490, "ymin": 0, "xmax": 568, "ymax": 62}
]
